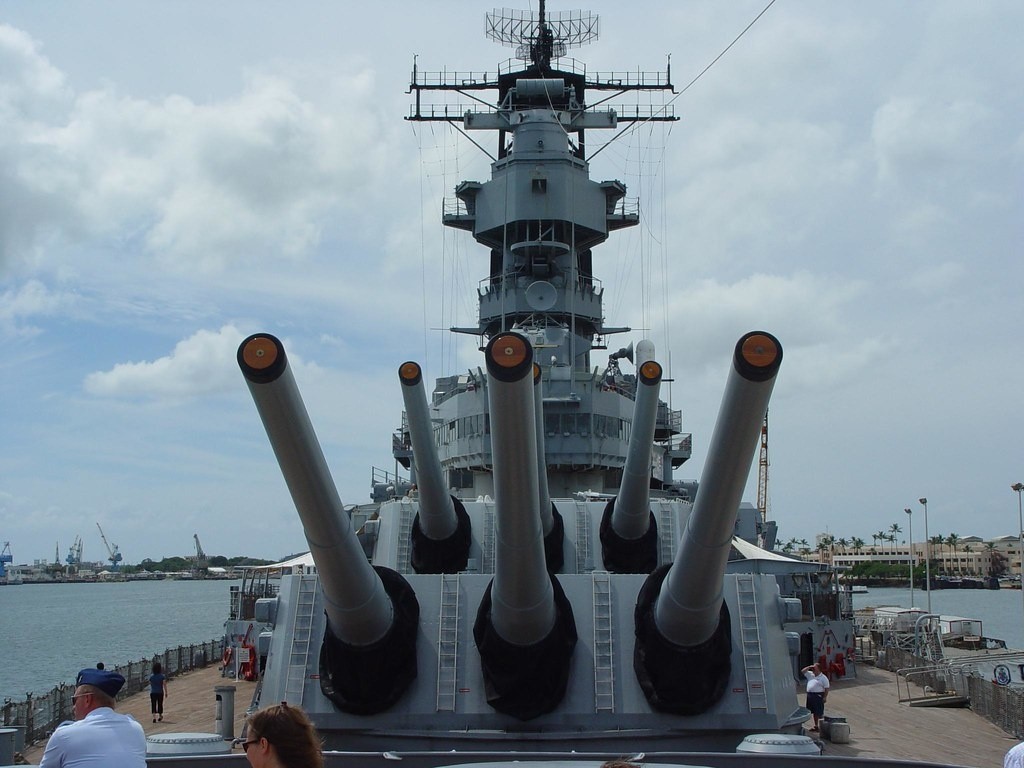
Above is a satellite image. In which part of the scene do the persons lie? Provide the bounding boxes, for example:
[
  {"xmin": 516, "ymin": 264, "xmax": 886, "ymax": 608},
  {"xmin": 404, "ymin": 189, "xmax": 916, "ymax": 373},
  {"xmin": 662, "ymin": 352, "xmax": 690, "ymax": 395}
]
[
  {"xmin": 801, "ymin": 662, "xmax": 831, "ymax": 732},
  {"xmin": 1003, "ymin": 742, "xmax": 1024, "ymax": 768},
  {"xmin": 149, "ymin": 663, "xmax": 167, "ymax": 723},
  {"xmin": 38, "ymin": 669, "xmax": 148, "ymax": 768},
  {"xmin": 408, "ymin": 484, "xmax": 417, "ymax": 497},
  {"xmin": 96, "ymin": 663, "xmax": 104, "ymax": 670},
  {"xmin": 242, "ymin": 700, "xmax": 324, "ymax": 768}
]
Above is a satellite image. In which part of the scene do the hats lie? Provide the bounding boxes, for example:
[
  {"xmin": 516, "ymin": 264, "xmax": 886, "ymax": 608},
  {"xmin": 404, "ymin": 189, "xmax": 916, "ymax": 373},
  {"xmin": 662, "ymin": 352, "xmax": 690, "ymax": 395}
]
[{"xmin": 76, "ymin": 669, "xmax": 126, "ymax": 698}]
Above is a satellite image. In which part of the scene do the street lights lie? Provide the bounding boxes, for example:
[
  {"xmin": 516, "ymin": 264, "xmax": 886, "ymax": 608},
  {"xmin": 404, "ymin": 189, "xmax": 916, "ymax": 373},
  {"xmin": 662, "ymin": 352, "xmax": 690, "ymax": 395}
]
[
  {"xmin": 904, "ymin": 508, "xmax": 915, "ymax": 607},
  {"xmin": 919, "ymin": 497, "xmax": 931, "ymax": 615},
  {"xmin": 1012, "ymin": 482, "xmax": 1023, "ymax": 605}
]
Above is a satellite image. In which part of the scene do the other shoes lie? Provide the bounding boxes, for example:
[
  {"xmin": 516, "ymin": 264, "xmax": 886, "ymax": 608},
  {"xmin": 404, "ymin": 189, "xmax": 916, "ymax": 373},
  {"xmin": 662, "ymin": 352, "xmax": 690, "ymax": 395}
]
[
  {"xmin": 808, "ymin": 726, "xmax": 818, "ymax": 731},
  {"xmin": 153, "ymin": 719, "xmax": 156, "ymax": 723},
  {"xmin": 158, "ymin": 717, "xmax": 162, "ymax": 721}
]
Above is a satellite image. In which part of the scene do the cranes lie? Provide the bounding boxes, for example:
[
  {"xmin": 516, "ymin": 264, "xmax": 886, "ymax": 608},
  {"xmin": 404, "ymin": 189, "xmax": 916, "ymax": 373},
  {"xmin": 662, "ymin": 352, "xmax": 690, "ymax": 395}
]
[
  {"xmin": 96, "ymin": 522, "xmax": 123, "ymax": 572},
  {"xmin": 192, "ymin": 533, "xmax": 209, "ymax": 575},
  {"xmin": 65, "ymin": 534, "xmax": 84, "ymax": 565},
  {"xmin": 54, "ymin": 541, "xmax": 62, "ymax": 565},
  {"xmin": 0, "ymin": 540, "xmax": 13, "ymax": 577}
]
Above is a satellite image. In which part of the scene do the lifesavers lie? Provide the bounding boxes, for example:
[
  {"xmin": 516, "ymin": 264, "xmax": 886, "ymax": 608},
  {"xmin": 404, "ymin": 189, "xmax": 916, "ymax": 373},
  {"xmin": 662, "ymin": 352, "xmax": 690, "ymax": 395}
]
[{"xmin": 222, "ymin": 648, "xmax": 232, "ymax": 666}]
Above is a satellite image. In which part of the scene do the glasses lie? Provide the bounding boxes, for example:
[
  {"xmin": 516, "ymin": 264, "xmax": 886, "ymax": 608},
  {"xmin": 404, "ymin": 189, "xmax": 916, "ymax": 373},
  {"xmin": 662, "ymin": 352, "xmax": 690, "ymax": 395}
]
[
  {"xmin": 242, "ymin": 740, "xmax": 258, "ymax": 752},
  {"xmin": 71, "ymin": 692, "xmax": 94, "ymax": 705}
]
